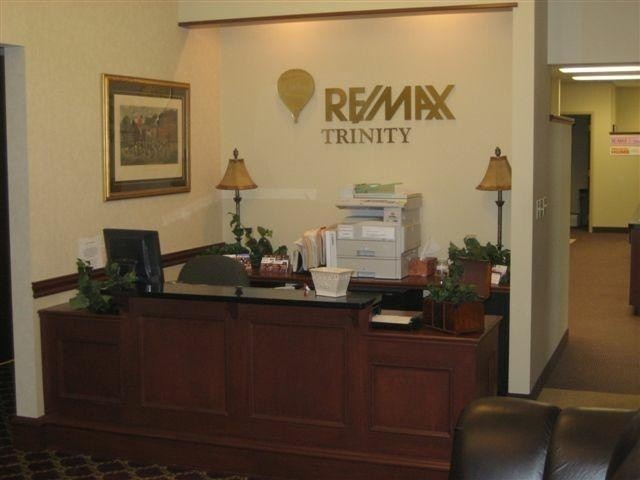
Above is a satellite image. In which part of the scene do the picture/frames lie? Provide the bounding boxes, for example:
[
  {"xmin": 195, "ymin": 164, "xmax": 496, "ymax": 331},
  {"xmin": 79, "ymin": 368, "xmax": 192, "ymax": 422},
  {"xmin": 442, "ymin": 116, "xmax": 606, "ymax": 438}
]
[{"xmin": 100, "ymin": 71, "xmax": 193, "ymax": 202}]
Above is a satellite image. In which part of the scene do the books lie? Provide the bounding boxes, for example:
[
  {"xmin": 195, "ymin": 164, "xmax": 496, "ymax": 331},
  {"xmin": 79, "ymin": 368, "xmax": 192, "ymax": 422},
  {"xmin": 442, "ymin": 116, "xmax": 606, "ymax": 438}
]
[{"xmin": 289, "ymin": 224, "xmax": 337, "ymax": 274}]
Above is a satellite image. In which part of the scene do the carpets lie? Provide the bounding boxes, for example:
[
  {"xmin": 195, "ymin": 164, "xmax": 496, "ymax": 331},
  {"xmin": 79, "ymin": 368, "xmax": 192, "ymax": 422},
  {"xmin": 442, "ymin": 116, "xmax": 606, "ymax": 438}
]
[{"xmin": 0, "ymin": 374, "xmax": 251, "ymax": 480}]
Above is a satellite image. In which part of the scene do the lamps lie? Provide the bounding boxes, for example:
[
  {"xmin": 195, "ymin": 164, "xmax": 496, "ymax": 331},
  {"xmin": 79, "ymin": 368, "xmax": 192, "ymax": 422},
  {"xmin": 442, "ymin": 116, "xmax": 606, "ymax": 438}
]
[
  {"xmin": 475, "ymin": 146, "xmax": 514, "ymax": 254},
  {"xmin": 215, "ymin": 148, "xmax": 258, "ymax": 246}
]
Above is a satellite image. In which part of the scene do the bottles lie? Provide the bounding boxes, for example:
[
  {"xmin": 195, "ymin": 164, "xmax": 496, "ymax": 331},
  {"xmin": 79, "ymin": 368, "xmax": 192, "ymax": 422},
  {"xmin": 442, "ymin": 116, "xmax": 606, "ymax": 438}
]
[{"xmin": 435, "ymin": 256, "xmax": 449, "ymax": 283}]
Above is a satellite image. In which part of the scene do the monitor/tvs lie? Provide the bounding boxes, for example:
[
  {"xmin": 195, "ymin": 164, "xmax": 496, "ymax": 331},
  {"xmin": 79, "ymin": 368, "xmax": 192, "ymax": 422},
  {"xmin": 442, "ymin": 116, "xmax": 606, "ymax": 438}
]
[{"xmin": 103, "ymin": 228, "xmax": 164, "ymax": 294}]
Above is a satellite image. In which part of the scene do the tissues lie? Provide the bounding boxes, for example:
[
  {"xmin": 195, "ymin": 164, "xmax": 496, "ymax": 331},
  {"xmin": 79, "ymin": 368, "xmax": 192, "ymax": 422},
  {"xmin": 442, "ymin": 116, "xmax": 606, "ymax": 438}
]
[{"xmin": 409, "ymin": 237, "xmax": 442, "ymax": 277}]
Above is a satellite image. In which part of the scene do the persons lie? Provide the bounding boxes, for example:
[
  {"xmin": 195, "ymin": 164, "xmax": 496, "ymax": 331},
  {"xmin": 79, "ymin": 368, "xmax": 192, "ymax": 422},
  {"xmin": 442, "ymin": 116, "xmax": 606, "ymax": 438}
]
[
  {"xmin": 280, "ymin": 262, "xmax": 287, "ymax": 272},
  {"xmin": 245, "ymin": 260, "xmax": 251, "ymax": 269}
]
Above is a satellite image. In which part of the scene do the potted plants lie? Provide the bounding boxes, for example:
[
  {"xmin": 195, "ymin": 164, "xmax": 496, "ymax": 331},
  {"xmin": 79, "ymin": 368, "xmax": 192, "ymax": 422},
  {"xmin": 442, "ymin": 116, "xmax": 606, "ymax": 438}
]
[
  {"xmin": 69, "ymin": 257, "xmax": 135, "ymax": 312},
  {"xmin": 423, "ymin": 235, "xmax": 512, "ymax": 334}
]
